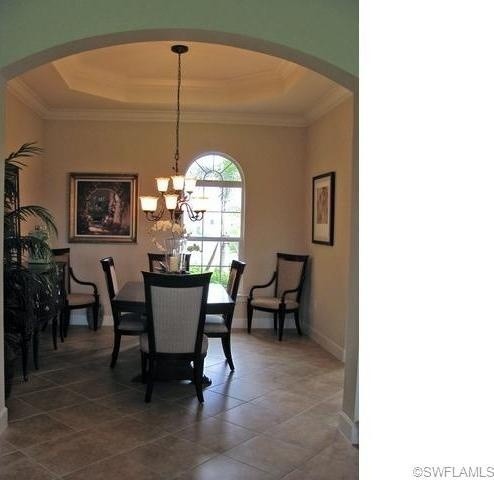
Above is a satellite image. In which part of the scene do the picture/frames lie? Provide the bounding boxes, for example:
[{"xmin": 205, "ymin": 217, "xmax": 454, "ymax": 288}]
[
  {"xmin": 66, "ymin": 171, "xmax": 139, "ymax": 244},
  {"xmin": 312, "ymin": 171, "xmax": 335, "ymax": 246},
  {"xmin": 5, "ymin": 163, "xmax": 21, "ymax": 271}
]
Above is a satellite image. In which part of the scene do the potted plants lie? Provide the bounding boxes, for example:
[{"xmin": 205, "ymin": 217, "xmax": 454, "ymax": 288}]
[{"xmin": 5, "ymin": 141, "xmax": 58, "ymax": 400}]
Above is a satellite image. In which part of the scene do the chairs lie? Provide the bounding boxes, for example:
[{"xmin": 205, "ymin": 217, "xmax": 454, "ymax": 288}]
[
  {"xmin": 50, "ymin": 248, "xmax": 99, "ymax": 338},
  {"xmin": 246, "ymin": 253, "xmax": 309, "ymax": 341},
  {"xmin": 139, "ymin": 270, "xmax": 213, "ymax": 403},
  {"xmin": 203, "ymin": 260, "xmax": 246, "ymax": 370},
  {"xmin": 148, "ymin": 253, "xmax": 191, "ymax": 272},
  {"xmin": 100, "ymin": 257, "xmax": 149, "ymax": 368}
]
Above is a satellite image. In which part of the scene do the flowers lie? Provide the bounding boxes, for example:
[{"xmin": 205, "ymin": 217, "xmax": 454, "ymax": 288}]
[{"xmin": 147, "ymin": 220, "xmax": 186, "ymax": 252}]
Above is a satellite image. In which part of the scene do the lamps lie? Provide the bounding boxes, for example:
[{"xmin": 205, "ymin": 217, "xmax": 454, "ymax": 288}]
[{"xmin": 138, "ymin": 44, "xmax": 209, "ymax": 223}]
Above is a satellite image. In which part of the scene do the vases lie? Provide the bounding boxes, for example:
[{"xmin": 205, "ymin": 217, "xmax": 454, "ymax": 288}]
[{"xmin": 164, "ymin": 237, "xmax": 188, "ymax": 274}]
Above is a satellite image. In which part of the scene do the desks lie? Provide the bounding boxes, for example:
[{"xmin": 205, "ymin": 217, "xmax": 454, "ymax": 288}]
[
  {"xmin": 4, "ymin": 262, "xmax": 64, "ymax": 382},
  {"xmin": 112, "ymin": 280, "xmax": 235, "ymax": 371}
]
